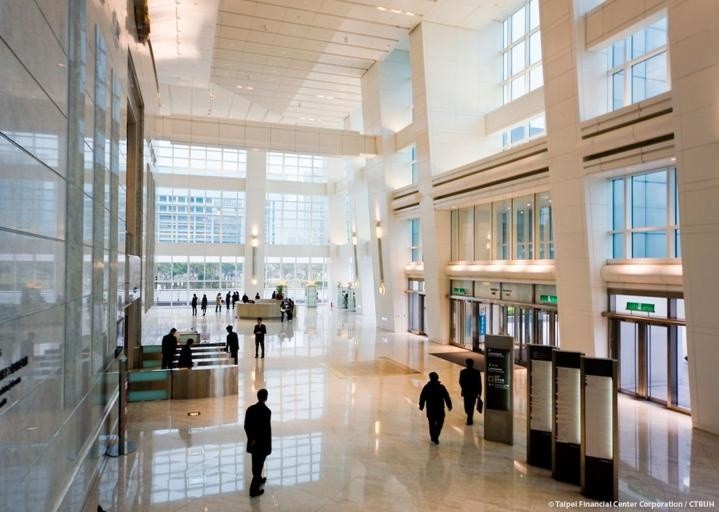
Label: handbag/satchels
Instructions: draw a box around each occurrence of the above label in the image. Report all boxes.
[476,397,483,413]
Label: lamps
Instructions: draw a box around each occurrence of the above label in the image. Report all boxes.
[251,228,258,285]
[352,222,360,289]
[375,203,386,295]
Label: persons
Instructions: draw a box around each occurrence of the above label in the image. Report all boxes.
[459,357,482,426]
[20,332,36,377]
[342,290,348,309]
[418,371,452,446]
[243,389,273,498]
[159,287,296,372]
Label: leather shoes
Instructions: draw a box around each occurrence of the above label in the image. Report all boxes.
[251,489,264,497]
[260,477,266,483]
